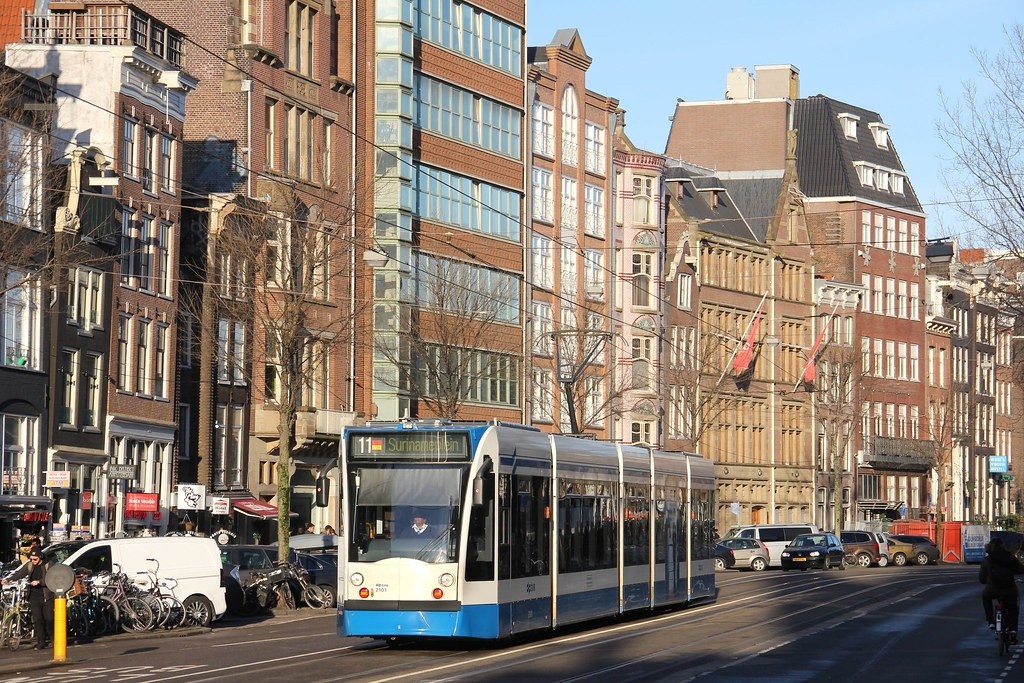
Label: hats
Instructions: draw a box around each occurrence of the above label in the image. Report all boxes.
[30,549,43,560]
[985,538,1005,553]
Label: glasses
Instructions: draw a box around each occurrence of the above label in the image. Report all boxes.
[30,557,39,562]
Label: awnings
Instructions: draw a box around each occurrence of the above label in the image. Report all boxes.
[231,499,299,520]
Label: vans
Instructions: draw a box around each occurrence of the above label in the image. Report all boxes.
[721,524,819,567]
[2,537,228,623]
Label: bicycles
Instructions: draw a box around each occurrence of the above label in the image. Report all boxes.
[989,575,1023,652]
[0,559,187,652]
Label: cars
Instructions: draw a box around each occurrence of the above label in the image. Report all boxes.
[830,530,916,568]
[268,534,346,567]
[979,541,1024,584]
[890,535,940,565]
[715,538,770,574]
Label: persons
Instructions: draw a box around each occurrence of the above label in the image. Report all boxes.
[32,538,42,549]
[325,525,336,535]
[1,547,55,651]
[979,537,1024,643]
[305,523,315,534]
[399,508,437,538]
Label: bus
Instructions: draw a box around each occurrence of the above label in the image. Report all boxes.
[316,329,716,638]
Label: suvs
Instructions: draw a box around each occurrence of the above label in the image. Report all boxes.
[781,533,846,572]
[217,545,338,607]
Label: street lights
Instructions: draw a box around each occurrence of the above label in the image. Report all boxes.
[656,164,693,457]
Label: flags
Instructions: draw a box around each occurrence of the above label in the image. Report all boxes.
[734,303,764,379]
[805,326,826,382]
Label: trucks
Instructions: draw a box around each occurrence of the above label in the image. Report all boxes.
[962,525,990,564]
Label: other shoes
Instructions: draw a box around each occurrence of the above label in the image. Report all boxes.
[988,623,995,629]
[1010,631,1018,645]
[33,640,47,651]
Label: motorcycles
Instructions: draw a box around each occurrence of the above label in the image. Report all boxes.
[245,560,328,611]
